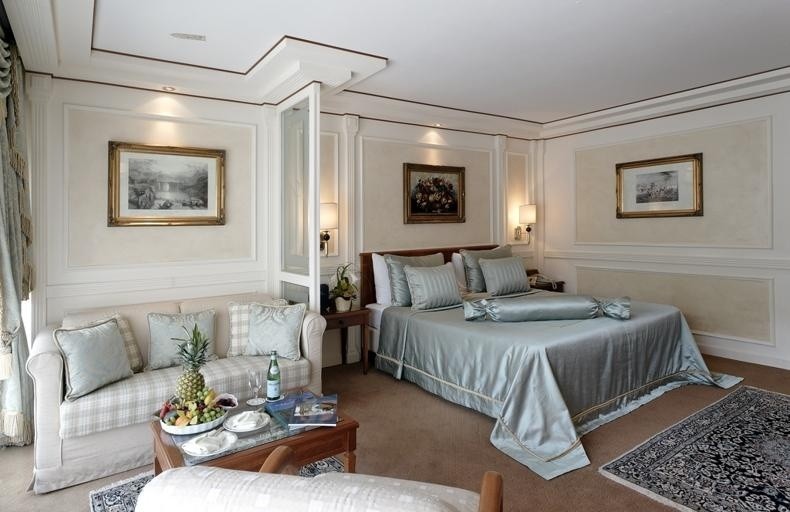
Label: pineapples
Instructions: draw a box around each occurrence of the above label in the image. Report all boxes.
[170,323,209,400]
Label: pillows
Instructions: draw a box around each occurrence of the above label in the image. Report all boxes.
[52,322,132,403]
[458,244,516,294]
[382,252,448,308]
[92,313,145,375]
[450,250,467,292]
[457,289,634,324]
[241,302,310,361]
[478,255,531,297]
[145,308,219,374]
[401,260,463,311]
[223,296,289,356]
[372,253,392,305]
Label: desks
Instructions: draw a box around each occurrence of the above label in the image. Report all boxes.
[530,279,565,293]
[319,308,373,377]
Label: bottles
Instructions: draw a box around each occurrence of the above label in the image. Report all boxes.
[266,349,282,401]
[320,283,330,316]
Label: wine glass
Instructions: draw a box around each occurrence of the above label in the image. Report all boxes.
[246,367,266,406]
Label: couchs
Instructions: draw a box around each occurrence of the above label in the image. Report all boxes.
[25,291,329,494]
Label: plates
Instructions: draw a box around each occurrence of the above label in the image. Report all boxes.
[181,429,237,458]
[265,395,285,402]
[221,411,271,433]
[160,409,229,435]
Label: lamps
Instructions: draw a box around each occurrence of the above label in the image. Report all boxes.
[512,203,539,247]
[319,204,338,256]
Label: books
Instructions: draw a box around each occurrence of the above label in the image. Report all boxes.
[265,392,318,431]
[287,393,338,427]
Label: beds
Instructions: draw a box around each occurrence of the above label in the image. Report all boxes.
[359,244,744,479]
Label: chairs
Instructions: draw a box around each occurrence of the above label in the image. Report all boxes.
[136,438,503,511]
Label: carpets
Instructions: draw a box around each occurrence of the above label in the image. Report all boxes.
[90,453,356,511]
[600,384,790,512]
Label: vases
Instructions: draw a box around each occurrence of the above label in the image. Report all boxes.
[335,299,353,314]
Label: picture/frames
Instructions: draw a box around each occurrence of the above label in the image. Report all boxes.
[106,140,228,230]
[615,151,704,220]
[402,162,466,223]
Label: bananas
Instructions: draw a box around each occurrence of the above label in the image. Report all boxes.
[203,389,216,405]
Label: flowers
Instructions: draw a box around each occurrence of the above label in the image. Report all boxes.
[330,262,360,300]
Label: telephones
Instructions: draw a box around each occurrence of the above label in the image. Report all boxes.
[531,273,555,283]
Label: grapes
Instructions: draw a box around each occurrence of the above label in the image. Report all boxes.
[199,407,227,424]
[196,388,209,400]
